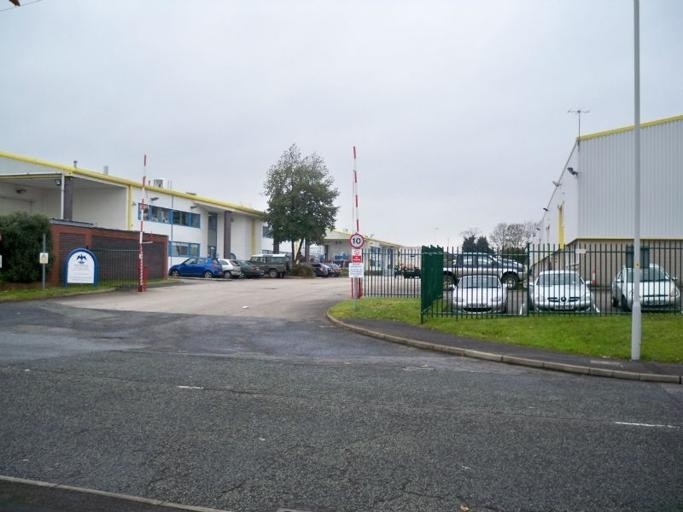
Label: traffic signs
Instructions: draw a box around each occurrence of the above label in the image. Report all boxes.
[348,232,364,249]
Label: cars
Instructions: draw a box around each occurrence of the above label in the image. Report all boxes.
[528,270,595,312]
[609,262,682,312]
[166,253,341,281]
[440,252,526,291]
[446,275,509,314]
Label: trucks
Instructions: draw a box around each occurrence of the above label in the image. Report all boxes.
[396,245,422,279]
